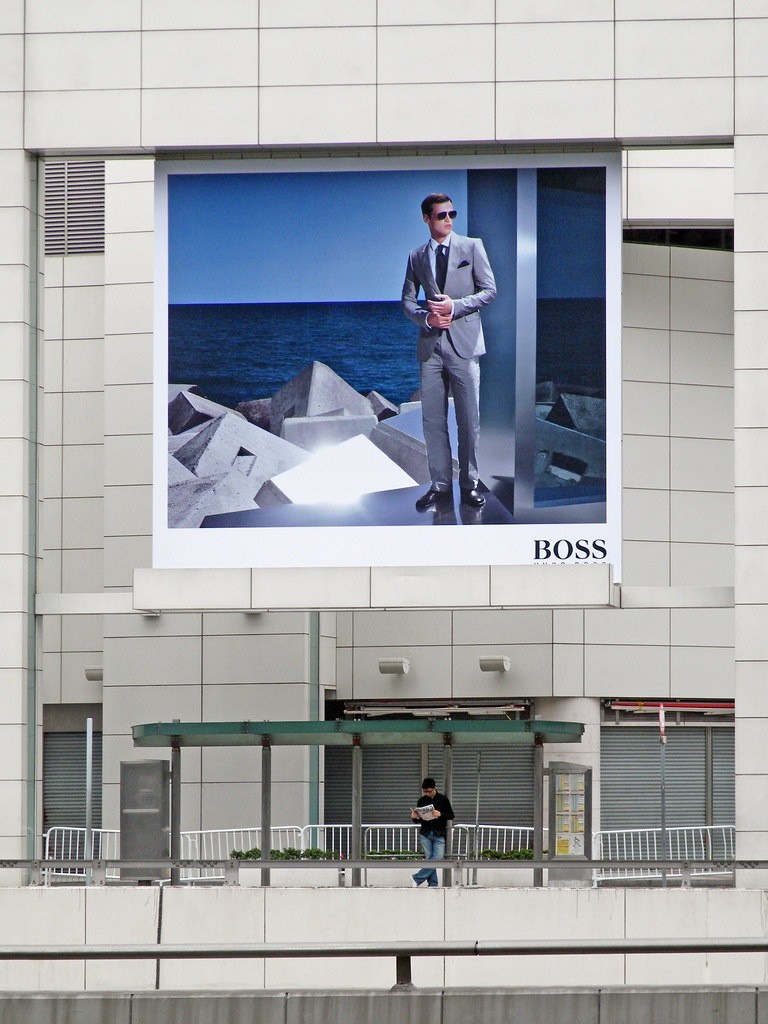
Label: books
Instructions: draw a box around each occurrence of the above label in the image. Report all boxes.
[410,804,438,821]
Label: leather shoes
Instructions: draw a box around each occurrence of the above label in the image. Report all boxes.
[417,490,449,507]
[462,487,486,506]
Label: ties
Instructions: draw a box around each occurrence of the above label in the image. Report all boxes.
[435,244,448,294]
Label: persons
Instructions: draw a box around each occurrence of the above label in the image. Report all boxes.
[409,777,455,887]
[402,192,498,511]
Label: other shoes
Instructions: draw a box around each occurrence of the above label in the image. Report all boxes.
[411,875,418,888]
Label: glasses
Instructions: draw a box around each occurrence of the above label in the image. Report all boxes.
[423,790,434,793]
[429,210,457,220]
